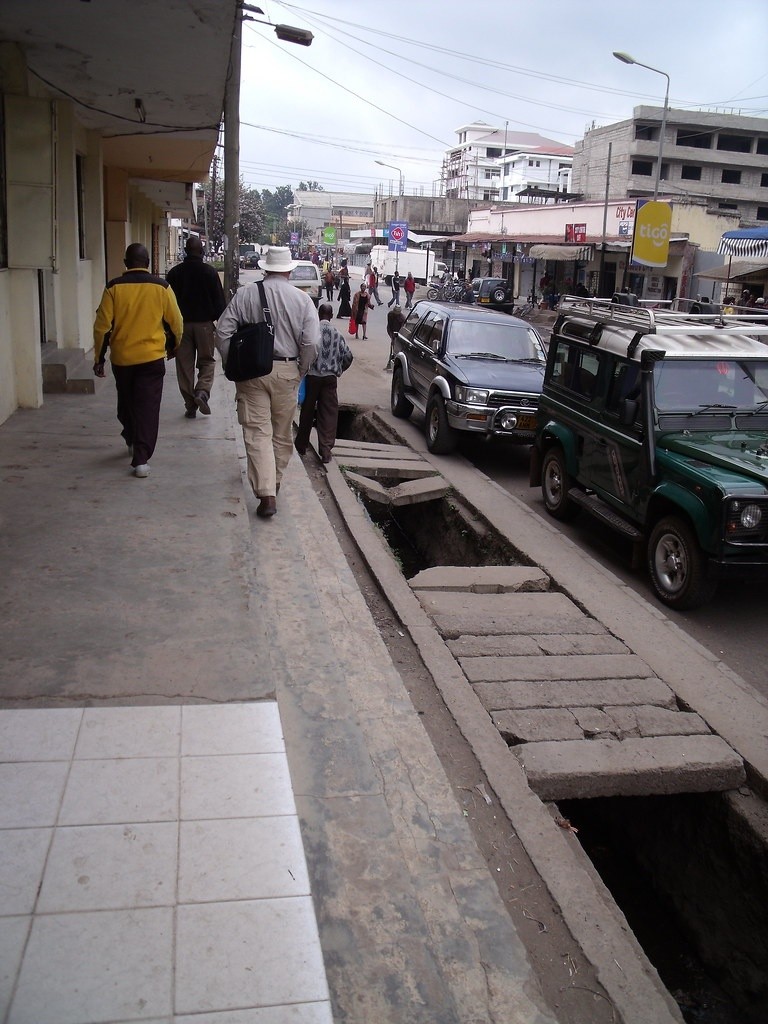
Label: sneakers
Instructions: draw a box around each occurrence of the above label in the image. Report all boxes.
[134,463,150,478]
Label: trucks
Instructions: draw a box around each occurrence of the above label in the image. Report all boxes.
[370,245,449,287]
[239,244,255,268]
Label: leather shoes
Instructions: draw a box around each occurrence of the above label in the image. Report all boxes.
[256,496,277,516]
[185,392,211,419]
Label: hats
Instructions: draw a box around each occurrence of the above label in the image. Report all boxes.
[408,272,411,275]
[258,246,298,272]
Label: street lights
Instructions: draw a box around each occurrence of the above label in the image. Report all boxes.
[613,51,670,308]
[224,15,314,309]
[374,161,401,271]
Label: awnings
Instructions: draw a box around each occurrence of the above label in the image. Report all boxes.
[529,245,594,261]
[716,226,768,258]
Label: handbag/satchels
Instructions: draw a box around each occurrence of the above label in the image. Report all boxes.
[224,281,275,382]
[348,319,357,334]
[298,376,306,406]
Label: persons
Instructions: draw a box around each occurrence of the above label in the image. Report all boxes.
[388,271,400,307]
[92,243,183,478]
[722,290,768,315]
[294,304,353,463]
[337,278,352,319]
[364,262,384,307]
[291,247,324,269]
[613,283,641,299]
[670,283,677,300]
[214,247,321,517]
[383,305,407,370]
[404,272,415,308]
[165,236,227,419]
[538,272,596,311]
[325,254,349,302]
[351,283,374,340]
[439,263,480,304]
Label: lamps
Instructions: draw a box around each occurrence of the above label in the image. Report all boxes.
[242,15,314,47]
[135,98,147,123]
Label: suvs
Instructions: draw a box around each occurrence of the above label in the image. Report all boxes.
[244,251,260,269]
[530,289,768,611]
[389,299,560,455]
[287,259,323,308]
[470,277,514,315]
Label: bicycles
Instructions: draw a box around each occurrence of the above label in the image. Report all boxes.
[427,279,471,303]
[514,295,535,318]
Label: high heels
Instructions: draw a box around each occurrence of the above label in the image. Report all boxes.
[356,333,359,339]
[363,336,368,340]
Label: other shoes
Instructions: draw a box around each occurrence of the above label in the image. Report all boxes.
[322,455,332,463]
[294,443,306,455]
[378,303,383,306]
